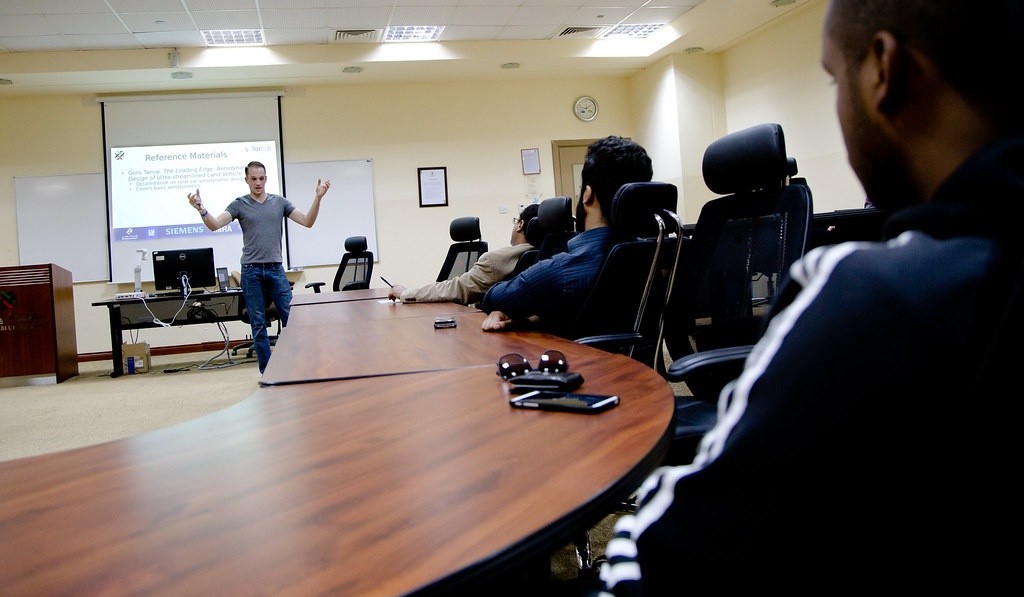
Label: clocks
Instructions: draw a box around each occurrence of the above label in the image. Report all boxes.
[574,96,599,122]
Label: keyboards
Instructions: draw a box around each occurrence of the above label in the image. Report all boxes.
[154,290,205,298]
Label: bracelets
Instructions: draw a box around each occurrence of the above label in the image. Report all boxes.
[201,210,208,221]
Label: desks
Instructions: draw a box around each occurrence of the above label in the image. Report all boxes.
[92,286,293,378]
[0,290,676,597]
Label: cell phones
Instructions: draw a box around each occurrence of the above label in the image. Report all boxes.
[434,316,457,328]
[510,391,621,415]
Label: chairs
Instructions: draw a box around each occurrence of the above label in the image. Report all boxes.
[503,123,899,585]
[436,217,488,283]
[305,237,374,294]
[232,271,294,358]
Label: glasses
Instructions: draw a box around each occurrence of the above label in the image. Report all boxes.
[496,349,568,379]
[512,218,519,225]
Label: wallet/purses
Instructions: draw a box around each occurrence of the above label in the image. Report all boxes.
[508,371,585,394]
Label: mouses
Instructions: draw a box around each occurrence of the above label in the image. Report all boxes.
[149,293,155,297]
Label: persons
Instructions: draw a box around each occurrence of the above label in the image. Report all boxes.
[388,204,540,304]
[188,161,330,376]
[598,0,1024,597]
[481,135,654,331]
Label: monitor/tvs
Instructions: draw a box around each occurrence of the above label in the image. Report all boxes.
[153,248,216,299]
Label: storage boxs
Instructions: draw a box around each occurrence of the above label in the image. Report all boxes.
[121,341,151,375]
